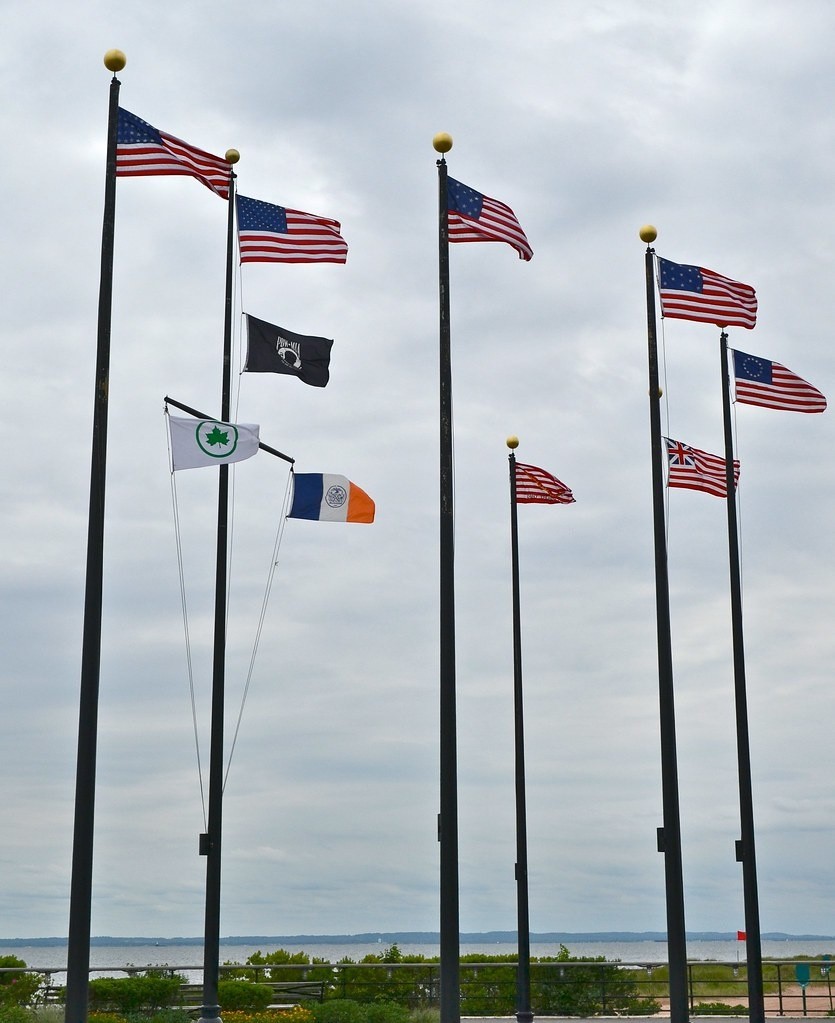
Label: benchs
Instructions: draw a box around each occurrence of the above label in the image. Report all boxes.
[34,986,65,1008]
[147,980,324,1011]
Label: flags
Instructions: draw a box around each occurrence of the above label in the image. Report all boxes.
[664,437,741,499]
[235,194,349,266]
[164,405,260,475]
[731,349,828,414]
[737,931,747,941]
[239,312,334,388]
[657,257,758,330]
[115,106,231,200]
[286,466,376,525]
[446,175,534,262]
[515,462,577,504]
